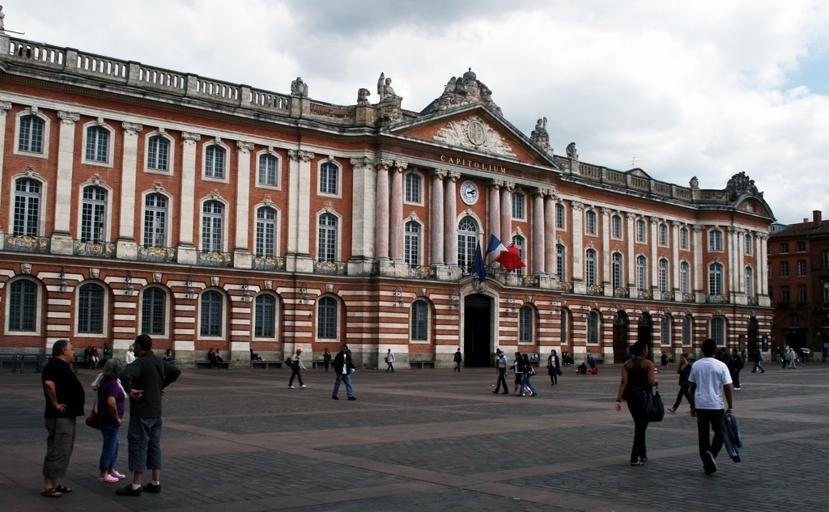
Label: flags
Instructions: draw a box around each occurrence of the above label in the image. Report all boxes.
[482,234,508,266]
[496,243,527,272]
[475,241,487,284]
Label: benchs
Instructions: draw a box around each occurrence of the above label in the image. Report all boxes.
[254,359,283,369]
[196,359,233,366]
[0,351,109,377]
[409,360,434,369]
[312,358,334,368]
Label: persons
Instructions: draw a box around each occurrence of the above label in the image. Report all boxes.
[96,357,127,484]
[716,337,801,390]
[660,350,670,369]
[215,348,224,363]
[323,348,331,372]
[453,348,462,372]
[666,353,696,413]
[577,362,587,375]
[588,365,599,376]
[207,347,217,368]
[492,348,509,394]
[546,349,561,386]
[508,351,524,394]
[384,348,395,373]
[165,348,174,362]
[563,350,574,364]
[687,338,734,476]
[332,344,358,400]
[125,344,136,368]
[287,348,307,389]
[115,334,182,496]
[517,354,538,397]
[251,348,262,361]
[85,342,112,368]
[40,339,86,498]
[587,350,595,364]
[614,340,658,467]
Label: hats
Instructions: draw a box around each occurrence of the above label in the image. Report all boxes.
[296,349,302,352]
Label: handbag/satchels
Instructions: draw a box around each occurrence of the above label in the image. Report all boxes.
[285,357,292,366]
[641,383,664,421]
[86,408,101,430]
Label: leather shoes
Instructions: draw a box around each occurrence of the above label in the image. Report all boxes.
[332,395,357,400]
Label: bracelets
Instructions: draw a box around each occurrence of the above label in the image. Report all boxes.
[616,399,621,403]
[726,408,734,413]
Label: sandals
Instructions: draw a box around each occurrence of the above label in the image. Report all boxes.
[40,484,72,497]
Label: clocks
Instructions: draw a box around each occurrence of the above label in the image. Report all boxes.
[459,179,480,206]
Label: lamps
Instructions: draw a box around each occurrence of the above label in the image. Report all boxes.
[393,286,404,309]
[299,284,311,305]
[122,271,135,298]
[56,265,69,296]
[240,280,253,304]
[505,294,517,316]
[184,275,196,302]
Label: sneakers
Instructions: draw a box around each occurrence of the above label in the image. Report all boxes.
[288,385,306,389]
[705,450,718,472]
[96,470,160,495]
[631,451,648,465]
